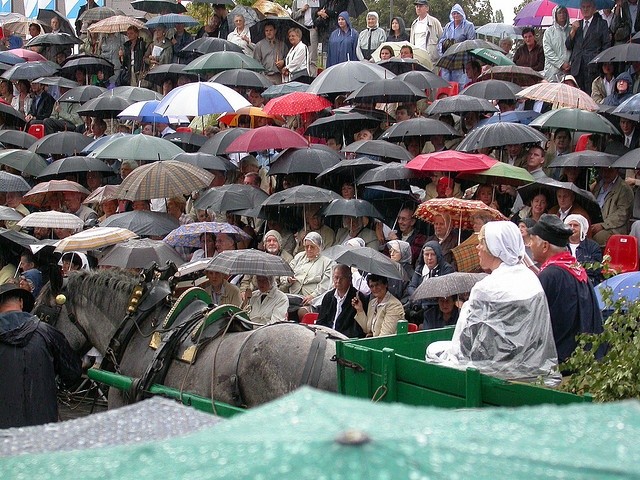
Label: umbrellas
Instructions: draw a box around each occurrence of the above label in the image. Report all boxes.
[205,248,295,299]
[305,53,398,93]
[252,0,289,18]
[627,31,640,43]
[82,184,122,204]
[423,94,500,114]
[129,0,188,14]
[223,123,312,153]
[469,110,543,127]
[304,112,383,157]
[457,160,536,204]
[404,150,499,189]
[356,161,419,191]
[470,48,517,67]
[435,39,508,70]
[153,73,253,135]
[513,0,585,27]
[98,210,180,236]
[454,112,548,162]
[16,211,85,239]
[22,180,91,208]
[215,106,274,127]
[262,183,346,233]
[250,15,311,62]
[370,41,433,71]
[515,179,605,225]
[36,148,114,183]
[609,148,640,169]
[0,12,26,23]
[0,386,640,480]
[31,75,82,89]
[527,98,623,153]
[315,156,389,198]
[267,134,347,176]
[79,135,114,153]
[456,71,525,100]
[551,0,616,21]
[547,143,621,191]
[393,65,455,90]
[476,22,526,40]
[7,48,48,61]
[116,92,190,136]
[587,35,640,73]
[593,271,640,311]
[313,197,385,218]
[378,60,435,75]
[53,225,140,254]
[262,92,334,127]
[85,126,186,160]
[378,109,465,155]
[164,125,210,147]
[0,127,39,148]
[77,6,116,20]
[197,125,253,154]
[0,50,27,66]
[57,80,106,102]
[1,17,45,35]
[611,92,640,122]
[413,196,511,231]
[144,63,205,88]
[61,55,114,86]
[111,153,215,213]
[37,8,75,36]
[98,80,165,102]
[77,89,130,134]
[476,64,544,86]
[194,182,270,210]
[162,221,253,257]
[226,4,266,32]
[409,270,492,315]
[0,226,40,250]
[181,44,266,73]
[261,82,311,96]
[0,101,27,126]
[59,53,115,67]
[451,233,483,273]
[320,0,367,18]
[0,59,63,80]
[0,205,24,221]
[515,82,601,112]
[179,31,245,55]
[28,130,96,154]
[321,246,410,303]
[86,15,148,61]
[170,151,240,172]
[343,72,427,128]
[192,0,235,27]
[206,61,276,89]
[341,138,416,162]
[0,170,32,191]
[24,31,85,47]
[0,149,50,177]
[143,13,200,39]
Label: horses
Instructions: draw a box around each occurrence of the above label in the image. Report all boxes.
[29,262,350,410]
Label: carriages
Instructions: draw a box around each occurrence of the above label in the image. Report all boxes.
[29,261,592,417]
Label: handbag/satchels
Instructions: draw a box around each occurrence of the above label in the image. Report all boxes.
[115,68,130,87]
[290,68,309,81]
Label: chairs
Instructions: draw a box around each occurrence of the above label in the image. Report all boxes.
[427,81,460,105]
[301,313,320,324]
[407,323,419,332]
[574,133,591,152]
[175,126,193,132]
[28,124,45,139]
[601,234,640,275]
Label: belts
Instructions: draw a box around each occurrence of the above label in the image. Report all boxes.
[305,26,315,29]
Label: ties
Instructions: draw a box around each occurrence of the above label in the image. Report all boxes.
[583,21,589,39]
[626,138,629,148]
[552,154,562,179]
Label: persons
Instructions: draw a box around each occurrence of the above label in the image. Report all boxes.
[0,191,7,206]
[428,88,449,102]
[19,251,48,283]
[55,228,72,239]
[5,191,30,217]
[336,215,380,252]
[246,89,264,109]
[119,25,148,86]
[166,195,195,225]
[340,180,355,199]
[162,76,176,96]
[316,1,348,51]
[143,23,173,66]
[28,23,41,39]
[215,232,238,253]
[425,220,562,380]
[424,171,463,200]
[591,62,617,102]
[226,14,252,43]
[48,193,65,212]
[0,79,14,100]
[4,35,23,51]
[409,0,444,64]
[547,188,592,239]
[378,46,395,60]
[24,80,57,122]
[196,209,211,222]
[251,229,293,294]
[177,74,192,86]
[436,3,476,91]
[564,0,611,95]
[626,61,640,94]
[4,165,15,174]
[11,80,32,113]
[282,27,311,83]
[599,9,613,26]
[525,145,550,182]
[237,115,251,128]
[97,238,184,270]
[527,213,608,377]
[239,155,276,193]
[464,61,483,87]
[476,184,499,210]
[502,144,527,168]
[64,192,99,230]
[87,171,104,190]
[30,86,85,136]
[287,232,337,321]
[430,134,448,152]
[157,121,177,136]
[0,252,17,283]
[585,134,604,152]
[120,159,138,181]
[211,4,231,39]
[398,208,427,269]
[75,67,89,85]
[562,215,603,287]
[560,168,588,190]
[591,167,635,254]
[141,124,157,136]
[614,117,640,152]
[75,0,100,37]
[472,215,494,232]
[387,239,415,279]
[497,98,516,111]
[386,16,411,42]
[294,204,336,255]
[399,44,414,59]
[226,210,256,236]
[282,174,297,189]
[95,68,108,87]
[1,112,20,130]
[190,232,219,261]
[118,123,132,134]
[542,5,575,82]
[33,227,51,239]
[435,175,456,199]
[351,273,405,337]
[518,217,538,261]
[97,199,119,223]
[623,169,640,219]
[252,23,289,84]
[19,269,45,298]
[477,147,495,158]
[354,129,373,142]
[90,117,108,139]
[423,295,461,329]
[291,1,321,80]
[545,128,572,179]
[50,17,63,33]
[355,11,388,60]
[407,241,455,300]
[326,134,343,152]
[521,193,562,225]
[205,270,243,308]
[425,213,459,242]
[499,37,515,60]
[0,283,84,428]
[396,104,414,122]
[55,52,67,65]
[560,75,579,89]
[132,200,148,210]
[61,253,83,277]
[195,11,222,39]
[611,0,640,43]
[513,28,545,71]
[602,72,636,107]
[240,274,289,324]
[267,213,294,254]
[243,172,262,189]
[317,264,368,339]
[326,10,360,67]
[170,23,194,65]
[403,136,420,154]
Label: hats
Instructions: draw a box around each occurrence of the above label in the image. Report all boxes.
[413,0,428,5]
[0,283,35,313]
[526,213,574,247]
